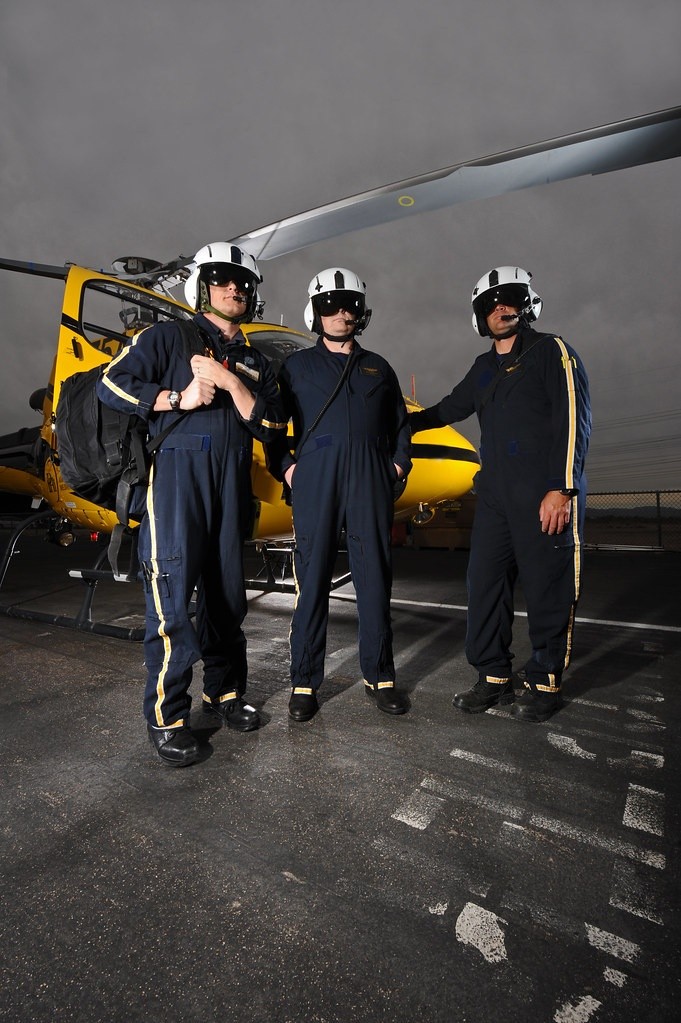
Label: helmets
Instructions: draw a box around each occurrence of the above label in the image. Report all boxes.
[470,265,543,338]
[184,241,264,318]
[304,267,372,342]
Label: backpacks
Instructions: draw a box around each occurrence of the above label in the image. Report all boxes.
[55,317,220,522]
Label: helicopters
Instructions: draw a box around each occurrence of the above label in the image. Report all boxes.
[0,104,681,641]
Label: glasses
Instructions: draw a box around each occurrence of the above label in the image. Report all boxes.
[311,291,365,318]
[472,285,525,318]
[200,265,258,297]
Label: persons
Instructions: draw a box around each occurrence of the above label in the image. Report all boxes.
[95,241,276,765]
[408,266,592,720]
[261,268,412,720]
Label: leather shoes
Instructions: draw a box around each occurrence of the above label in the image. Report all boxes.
[509,684,563,723]
[147,721,202,767]
[287,680,317,721]
[451,676,516,714]
[364,680,408,715]
[202,691,261,732]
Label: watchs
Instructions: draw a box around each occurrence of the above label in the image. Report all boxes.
[167,389,182,412]
[560,489,573,496]
